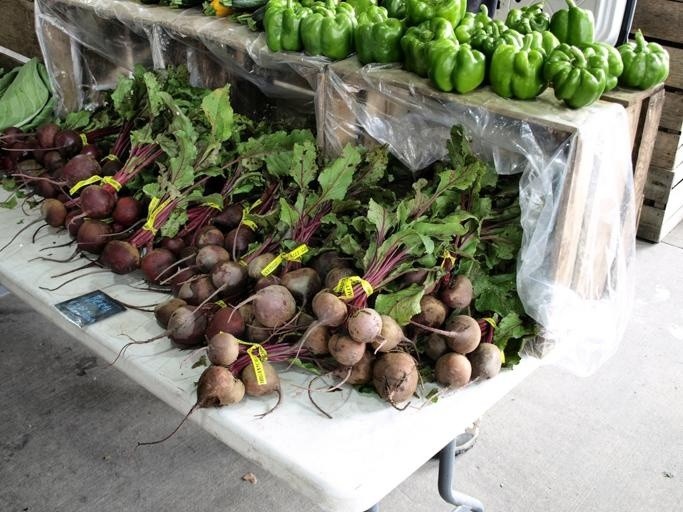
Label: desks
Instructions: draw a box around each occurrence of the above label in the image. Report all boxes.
[1,181,535,512]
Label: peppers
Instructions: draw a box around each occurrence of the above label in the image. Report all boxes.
[263,0,669,110]
